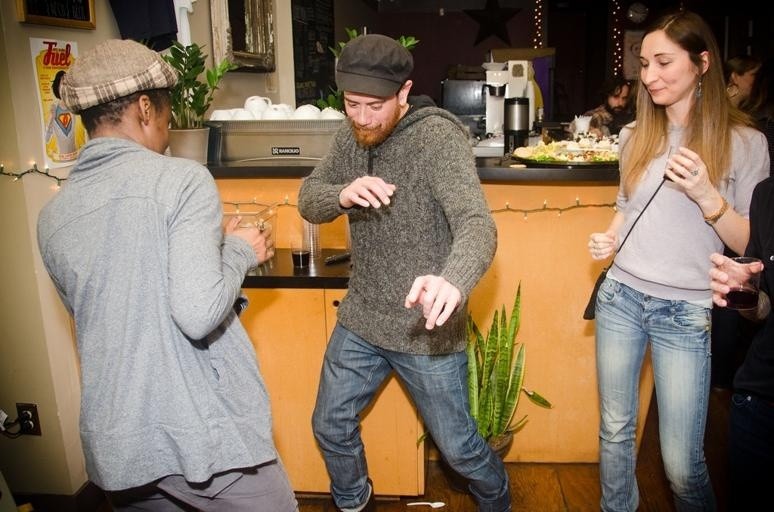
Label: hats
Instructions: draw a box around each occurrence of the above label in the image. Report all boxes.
[335,33,417,99]
[61,37,180,114]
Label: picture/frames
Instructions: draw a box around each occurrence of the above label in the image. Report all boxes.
[209,0,277,77]
[15,0,96,33]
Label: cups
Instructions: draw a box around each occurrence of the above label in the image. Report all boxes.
[291,238,313,272]
[207,95,346,119]
[714,256,766,314]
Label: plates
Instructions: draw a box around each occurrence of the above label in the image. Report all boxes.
[510,153,620,165]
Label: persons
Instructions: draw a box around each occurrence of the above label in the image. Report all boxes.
[708,175,774,512]
[588,12,770,512]
[581,52,774,176]
[296,34,498,512]
[38,39,300,512]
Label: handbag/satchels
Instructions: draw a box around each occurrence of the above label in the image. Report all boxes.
[583,117,705,322]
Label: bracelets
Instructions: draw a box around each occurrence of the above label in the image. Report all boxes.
[704,197,730,225]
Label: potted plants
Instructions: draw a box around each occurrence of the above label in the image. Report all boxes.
[411,280,553,496]
[159,41,236,169]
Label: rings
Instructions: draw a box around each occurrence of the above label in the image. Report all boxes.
[256,220,265,232]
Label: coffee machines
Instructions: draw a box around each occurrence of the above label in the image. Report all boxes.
[473,59,545,158]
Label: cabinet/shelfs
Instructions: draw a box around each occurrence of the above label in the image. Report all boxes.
[225,287,431,502]
